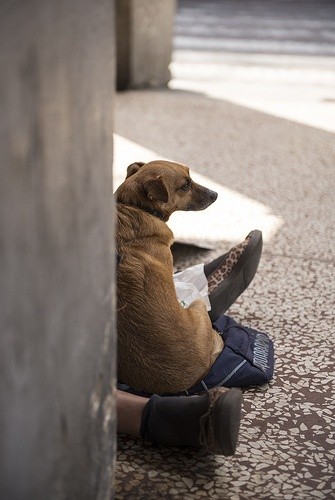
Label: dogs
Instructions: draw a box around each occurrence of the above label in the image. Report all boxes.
[115,160,224,395]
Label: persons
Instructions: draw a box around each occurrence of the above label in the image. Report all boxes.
[116,230,263,456]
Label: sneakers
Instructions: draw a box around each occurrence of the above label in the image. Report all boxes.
[201,384,244,457]
[207,229,262,322]
